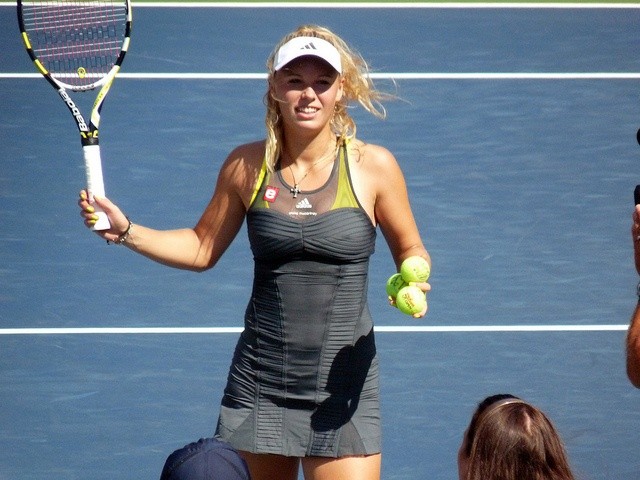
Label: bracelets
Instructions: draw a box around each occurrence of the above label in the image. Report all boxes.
[114,217,134,246]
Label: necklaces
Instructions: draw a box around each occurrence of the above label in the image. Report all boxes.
[282,146,337,198]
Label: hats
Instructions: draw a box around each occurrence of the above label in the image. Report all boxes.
[272,36,342,75]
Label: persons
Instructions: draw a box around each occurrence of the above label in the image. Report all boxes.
[455,393,577,479]
[624,127,640,391]
[72,25,435,480]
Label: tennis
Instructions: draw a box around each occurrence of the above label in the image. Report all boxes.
[396,285,425,314]
[386,273,406,299]
[400,255,430,285]
